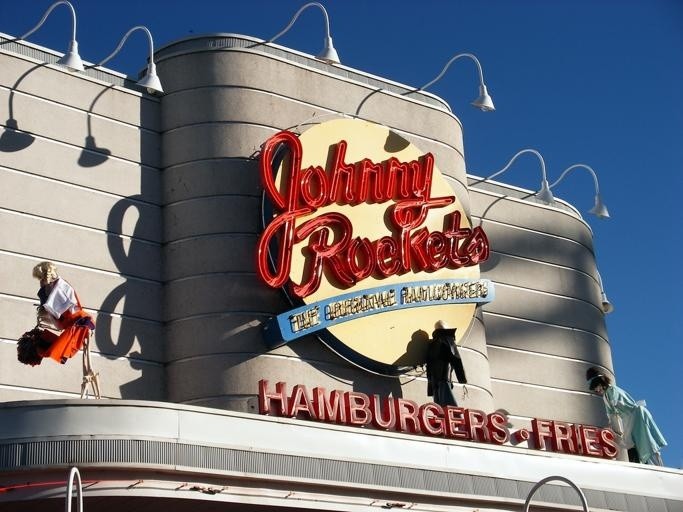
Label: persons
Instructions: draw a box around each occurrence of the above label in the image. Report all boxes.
[587,366,668,464]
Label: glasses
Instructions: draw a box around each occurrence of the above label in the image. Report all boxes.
[268,2,341,65]
[536,164,610,219]
[522,476,587,511]
[96,24,164,96]
[65,466,83,512]
[19,1,86,72]
[487,149,556,205]
[598,270,613,314]
[420,53,496,113]
